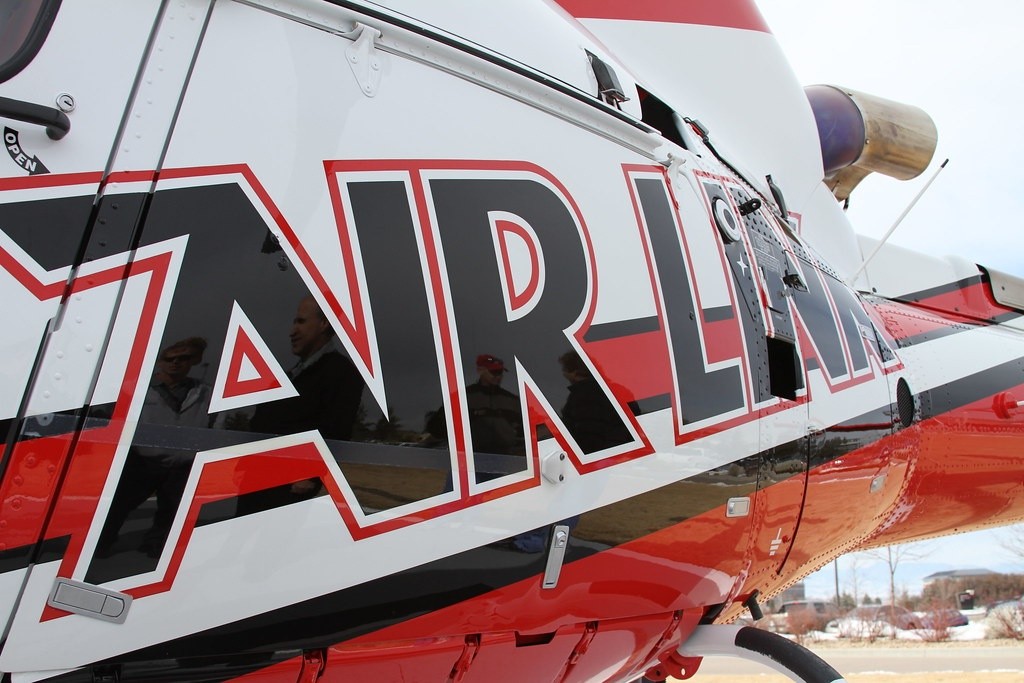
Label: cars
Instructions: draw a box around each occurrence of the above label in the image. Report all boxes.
[826,604,922,636]
[924,610,969,628]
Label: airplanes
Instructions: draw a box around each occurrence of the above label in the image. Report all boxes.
[1,1,1022,683]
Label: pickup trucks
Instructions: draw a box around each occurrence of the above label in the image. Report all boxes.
[765,599,844,635]
